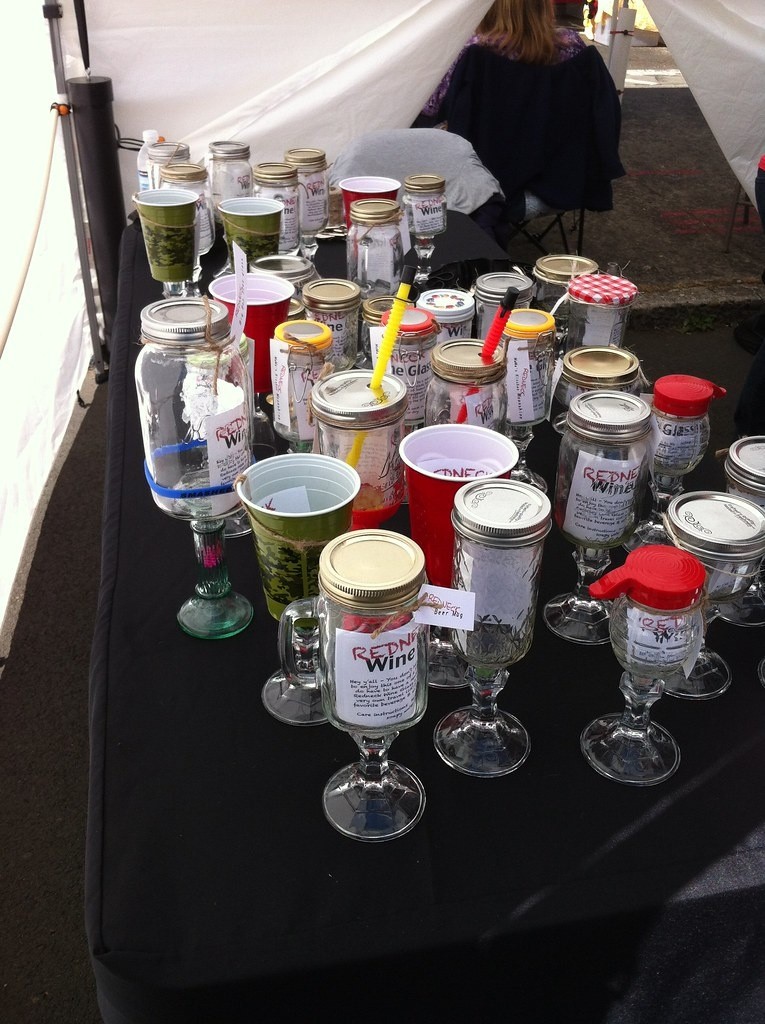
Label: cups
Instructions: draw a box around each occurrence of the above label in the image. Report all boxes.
[338,176,402,257]
[132,188,200,281]
[399,424,519,587]
[218,197,285,271]
[210,272,294,393]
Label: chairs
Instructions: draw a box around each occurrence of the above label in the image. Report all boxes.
[437,44,627,257]
[329,126,508,249]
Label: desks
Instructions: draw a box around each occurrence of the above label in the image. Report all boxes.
[83,210,765,1024]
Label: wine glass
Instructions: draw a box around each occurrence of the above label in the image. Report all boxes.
[134,142,765,842]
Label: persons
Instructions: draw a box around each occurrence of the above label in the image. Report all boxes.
[419,0,588,241]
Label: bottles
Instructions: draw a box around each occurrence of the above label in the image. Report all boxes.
[136,130,159,192]
[346,199,404,298]
